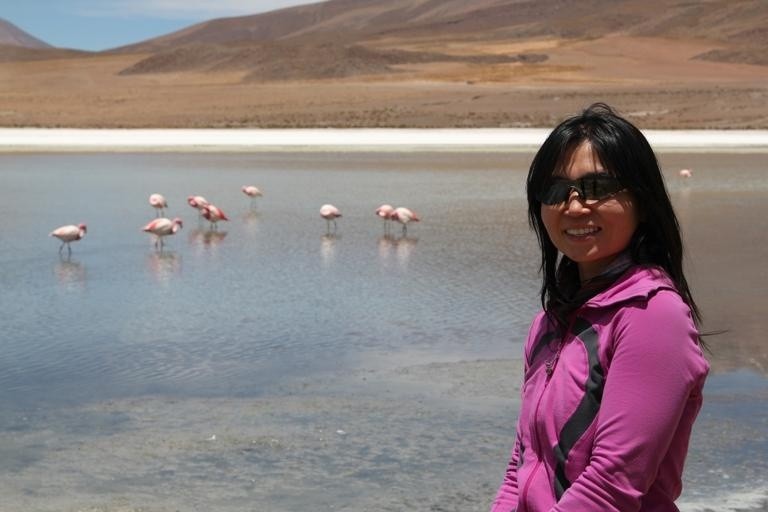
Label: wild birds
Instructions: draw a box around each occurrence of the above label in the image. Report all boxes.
[140,218,183,247]
[49,223,87,253]
[390,207,420,233]
[201,205,230,228]
[242,185,262,207]
[375,204,394,229]
[680,169,691,178]
[148,193,168,217]
[320,204,343,228]
[188,195,211,214]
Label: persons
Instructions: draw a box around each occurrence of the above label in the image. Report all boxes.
[492,99,711,512]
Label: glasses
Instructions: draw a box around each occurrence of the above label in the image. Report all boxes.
[533,172,624,205]
